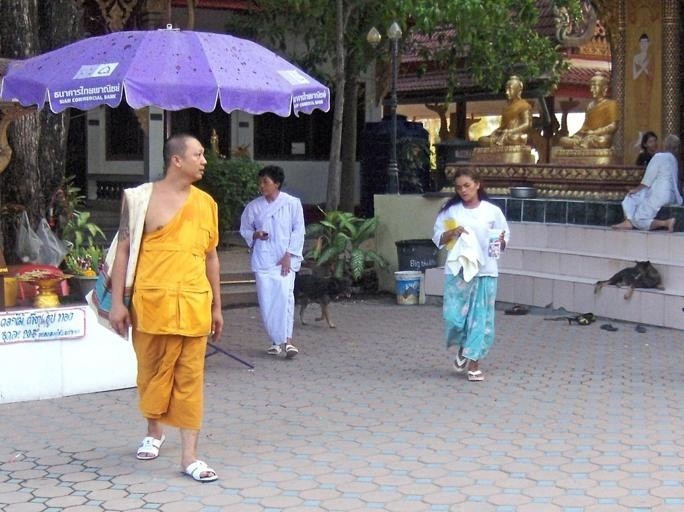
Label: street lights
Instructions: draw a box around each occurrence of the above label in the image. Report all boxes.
[365,21,403,195]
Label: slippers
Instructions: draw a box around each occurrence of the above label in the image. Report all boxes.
[266,345,282,355]
[178,460,218,481]
[137,434,165,460]
[286,344,298,357]
[505,306,528,314]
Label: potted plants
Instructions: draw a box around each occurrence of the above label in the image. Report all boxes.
[319,211,389,294]
[306,212,359,293]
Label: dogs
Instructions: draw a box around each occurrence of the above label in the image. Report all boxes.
[594,260,665,300]
[294,271,353,328]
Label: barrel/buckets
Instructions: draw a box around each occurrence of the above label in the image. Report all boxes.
[393,270,422,306]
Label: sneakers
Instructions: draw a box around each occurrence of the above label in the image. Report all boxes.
[635,323,646,332]
[600,324,618,331]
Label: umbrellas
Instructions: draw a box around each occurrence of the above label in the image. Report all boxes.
[0,23,331,142]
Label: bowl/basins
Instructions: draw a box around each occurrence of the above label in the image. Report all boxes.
[510,187,536,199]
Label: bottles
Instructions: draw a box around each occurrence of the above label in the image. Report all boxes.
[488,230,501,261]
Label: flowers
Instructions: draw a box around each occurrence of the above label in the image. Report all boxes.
[65,245,109,277]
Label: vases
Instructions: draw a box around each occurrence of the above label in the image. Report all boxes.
[77,275,98,304]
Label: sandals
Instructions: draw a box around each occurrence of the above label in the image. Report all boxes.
[467,370,484,381]
[454,349,467,371]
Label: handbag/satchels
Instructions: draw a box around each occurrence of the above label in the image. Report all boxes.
[85,181,153,340]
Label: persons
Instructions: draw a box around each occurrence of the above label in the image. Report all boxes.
[632,34,655,147]
[612,135,684,232]
[558,71,622,149]
[636,131,658,166]
[432,167,511,381]
[239,166,306,357]
[108,133,223,482]
[477,74,533,145]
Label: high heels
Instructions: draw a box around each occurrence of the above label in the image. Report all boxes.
[567,313,596,325]
